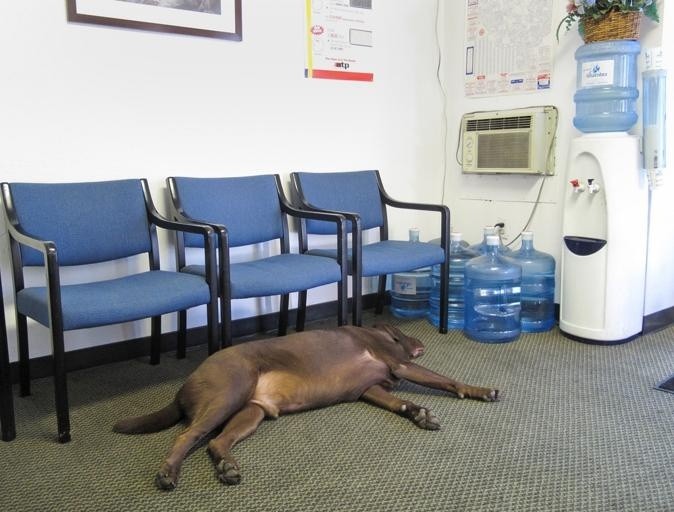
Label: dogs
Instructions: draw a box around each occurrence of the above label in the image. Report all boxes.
[111,323,503,492]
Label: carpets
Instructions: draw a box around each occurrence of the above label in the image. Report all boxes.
[653,373,674,394]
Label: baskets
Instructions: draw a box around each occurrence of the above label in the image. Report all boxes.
[585,11,640,43]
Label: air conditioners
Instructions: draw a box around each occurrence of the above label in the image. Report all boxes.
[460,106,555,175]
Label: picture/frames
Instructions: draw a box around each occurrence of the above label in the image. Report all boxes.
[66,0,242,42]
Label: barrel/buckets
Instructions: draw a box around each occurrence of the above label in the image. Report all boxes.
[391,225,556,342]
[572,40,642,132]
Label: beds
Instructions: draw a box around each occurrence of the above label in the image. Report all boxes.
[166,174,348,360]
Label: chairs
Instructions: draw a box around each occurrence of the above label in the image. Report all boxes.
[1,178,220,443]
[291,170,450,331]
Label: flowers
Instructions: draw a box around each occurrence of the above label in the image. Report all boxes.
[557,0,659,43]
